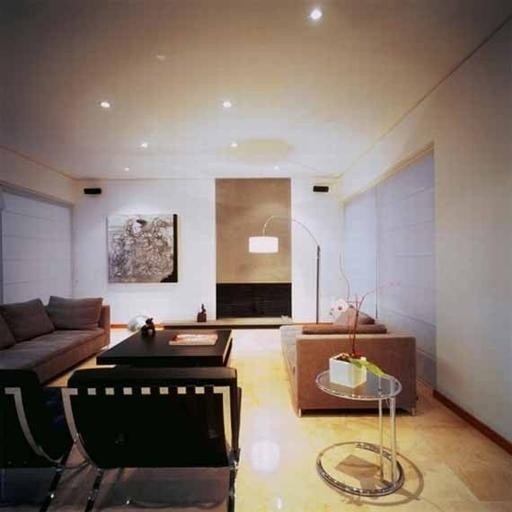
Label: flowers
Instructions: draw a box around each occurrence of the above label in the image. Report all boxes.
[328,282,382,354]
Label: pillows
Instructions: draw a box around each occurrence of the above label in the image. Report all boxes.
[0,314,17,350]
[45,296,104,330]
[301,322,387,334]
[333,306,375,325]
[0,297,55,343]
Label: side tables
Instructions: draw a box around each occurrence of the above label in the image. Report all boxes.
[315,368,405,497]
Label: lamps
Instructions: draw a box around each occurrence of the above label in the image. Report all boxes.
[249,214,320,323]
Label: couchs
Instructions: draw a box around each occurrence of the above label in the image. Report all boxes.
[279,310,416,417]
[0,304,111,386]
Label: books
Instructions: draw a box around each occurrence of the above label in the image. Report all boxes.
[168,334,218,346]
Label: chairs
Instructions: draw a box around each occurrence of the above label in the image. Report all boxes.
[61,365,242,512]
[0,367,74,512]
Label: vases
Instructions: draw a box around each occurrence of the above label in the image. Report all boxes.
[328,351,368,389]
